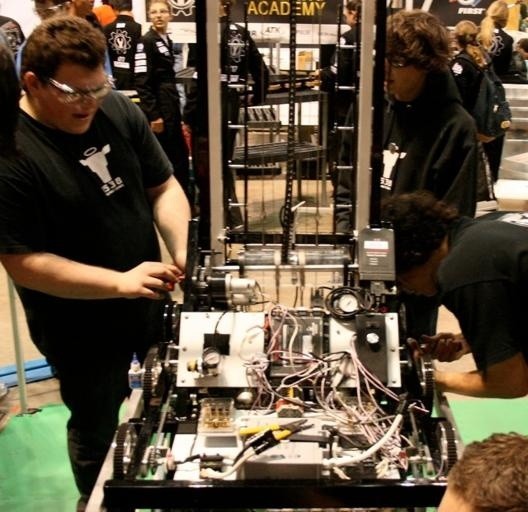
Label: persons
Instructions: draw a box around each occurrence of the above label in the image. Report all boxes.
[1,15,192,511]
[435,431,527,511]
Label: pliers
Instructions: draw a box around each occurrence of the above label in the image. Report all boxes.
[238,419,314,441]
